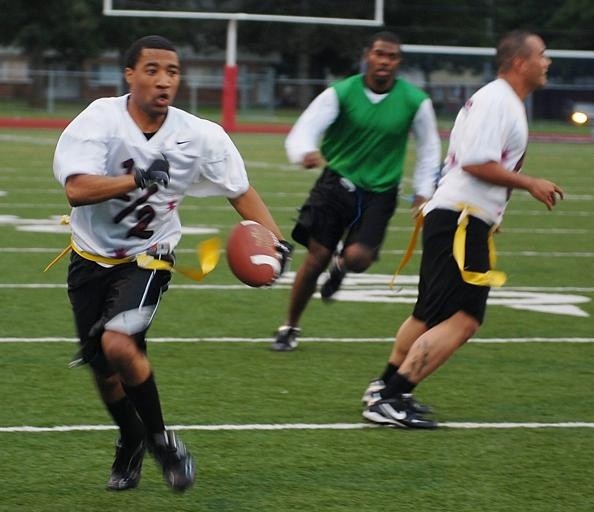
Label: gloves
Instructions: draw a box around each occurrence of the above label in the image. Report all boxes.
[134,158,172,190]
[274,238,293,275]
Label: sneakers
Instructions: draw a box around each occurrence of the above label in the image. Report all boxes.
[361,379,437,429]
[107,425,146,492]
[148,428,194,493]
[322,252,345,299]
[273,324,301,351]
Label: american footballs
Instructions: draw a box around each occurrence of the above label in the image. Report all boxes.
[227,219,282,288]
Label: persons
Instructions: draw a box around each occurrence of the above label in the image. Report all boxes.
[265,30,443,352]
[361,29,565,432]
[53,34,293,493]
